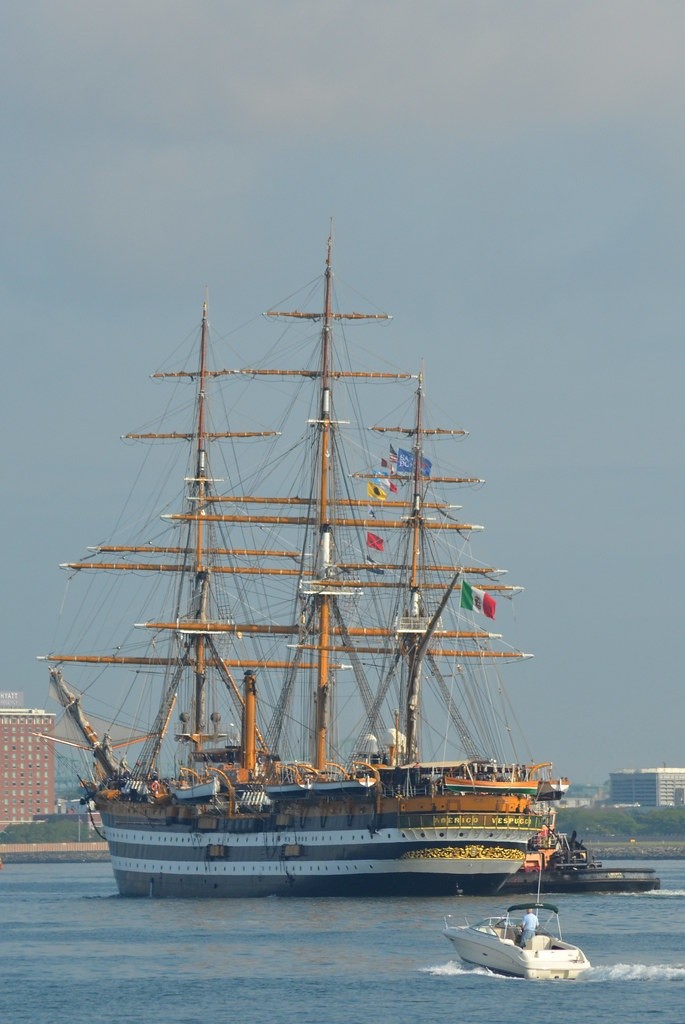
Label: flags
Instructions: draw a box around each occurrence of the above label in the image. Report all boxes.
[390,443,395,459]
[366,506,373,515]
[374,472,398,493]
[461,580,496,621]
[381,457,394,472]
[366,556,383,575]
[367,481,387,501]
[397,449,432,477]
[366,533,386,551]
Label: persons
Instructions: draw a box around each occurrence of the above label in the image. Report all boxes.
[519,909,540,948]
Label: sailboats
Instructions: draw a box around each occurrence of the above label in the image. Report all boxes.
[32,213,572,891]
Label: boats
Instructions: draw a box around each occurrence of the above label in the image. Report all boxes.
[501,834,662,894]
[440,860,593,983]
